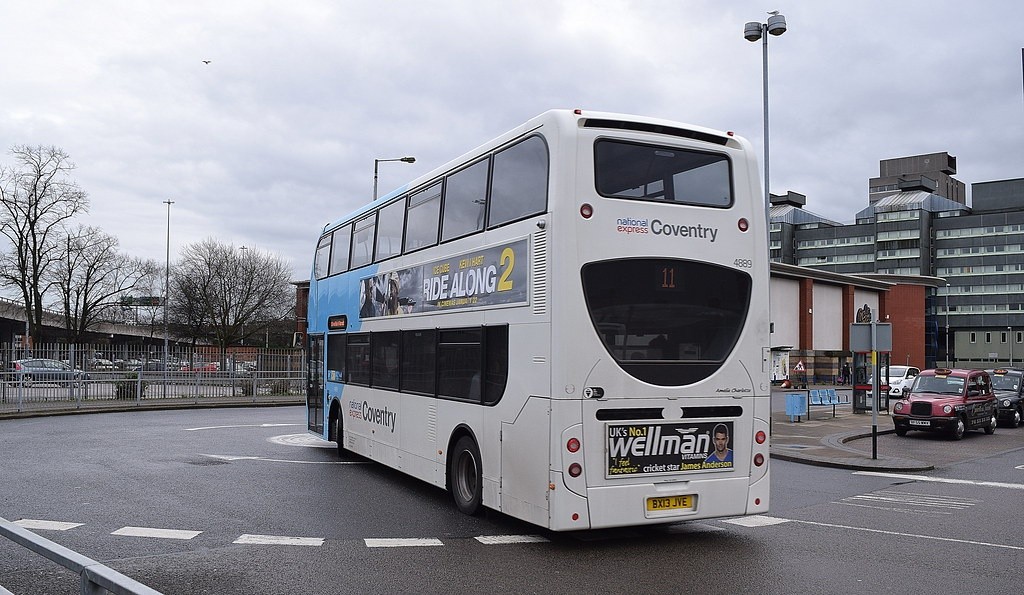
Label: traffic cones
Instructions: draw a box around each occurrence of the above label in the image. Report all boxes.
[780,379,793,389]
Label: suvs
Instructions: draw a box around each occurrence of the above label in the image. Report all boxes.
[980,368,1024,428]
[893,367,997,440]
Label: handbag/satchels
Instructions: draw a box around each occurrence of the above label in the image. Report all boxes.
[837,377,843,383]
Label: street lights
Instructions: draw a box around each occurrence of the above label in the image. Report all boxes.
[744,10,789,258]
[162,198,175,399]
[372,157,417,202]
[946,283,951,369]
[1007,326,1012,366]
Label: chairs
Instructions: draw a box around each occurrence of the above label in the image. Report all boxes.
[810,388,851,405]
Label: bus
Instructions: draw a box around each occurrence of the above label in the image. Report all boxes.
[291,107,769,533]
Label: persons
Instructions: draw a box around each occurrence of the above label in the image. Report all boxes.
[837,362,850,386]
[704,424,733,464]
[360,272,412,318]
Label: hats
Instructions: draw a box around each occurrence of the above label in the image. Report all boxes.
[389,272,400,294]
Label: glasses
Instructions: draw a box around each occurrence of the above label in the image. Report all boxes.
[366,286,373,292]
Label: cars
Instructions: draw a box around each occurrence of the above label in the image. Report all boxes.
[8,359,91,389]
[865,365,920,400]
[61,358,257,376]
